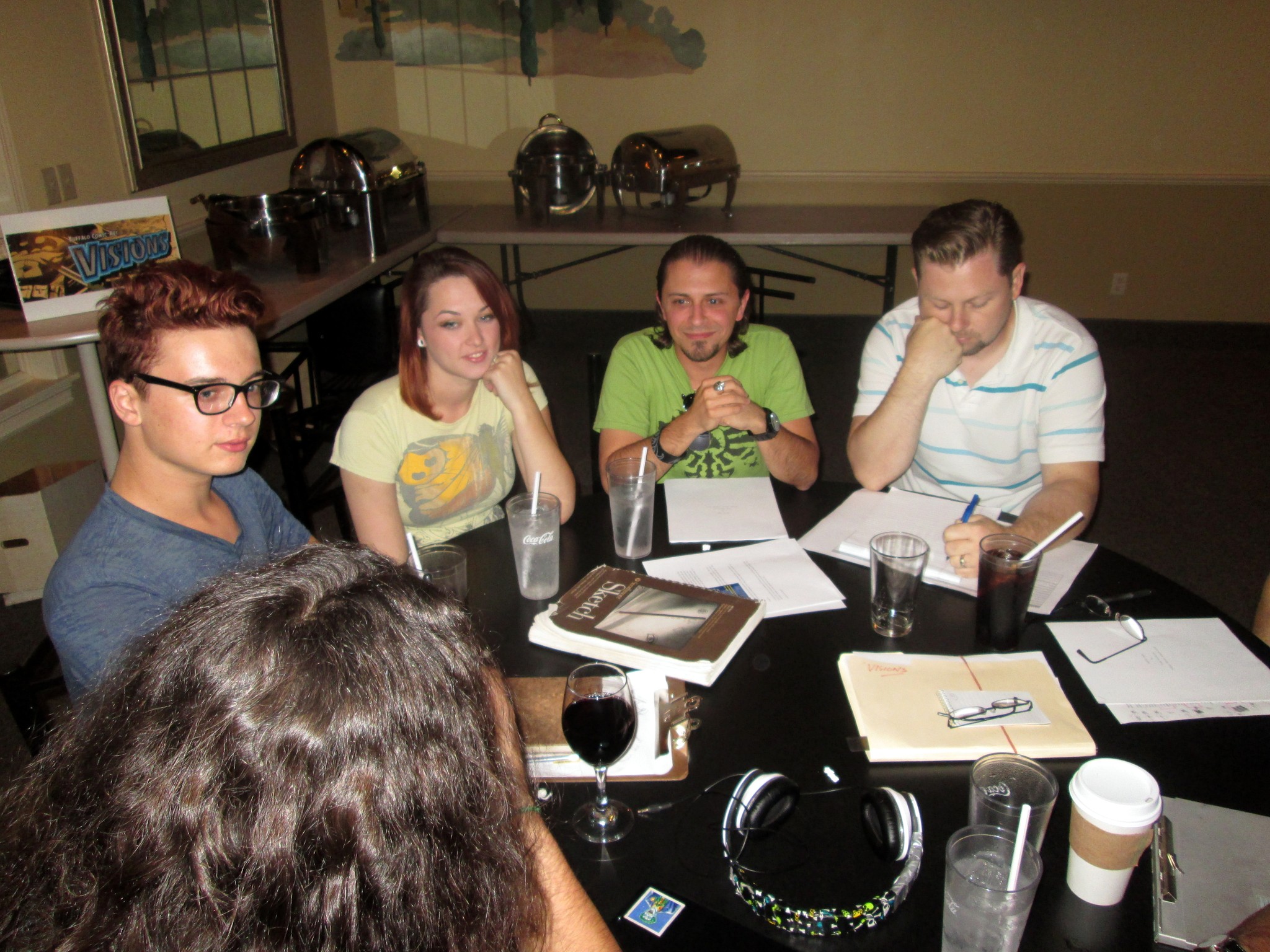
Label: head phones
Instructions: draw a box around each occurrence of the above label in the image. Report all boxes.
[720,767,924,935]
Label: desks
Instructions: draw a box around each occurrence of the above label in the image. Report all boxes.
[426,480,1270,952]
[436,203,945,332]
[0,204,471,479]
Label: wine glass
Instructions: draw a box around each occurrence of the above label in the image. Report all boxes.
[561,662,637,843]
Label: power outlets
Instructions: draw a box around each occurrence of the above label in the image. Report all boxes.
[1110,272,1129,298]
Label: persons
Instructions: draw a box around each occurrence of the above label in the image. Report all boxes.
[847,199,1107,578]
[593,233,820,492]
[328,247,576,568]
[0,537,622,952]
[1253,575,1270,646]
[1192,901,1270,952]
[39,259,321,711]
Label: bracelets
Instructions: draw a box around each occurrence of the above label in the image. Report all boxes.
[651,422,687,464]
[514,804,544,816]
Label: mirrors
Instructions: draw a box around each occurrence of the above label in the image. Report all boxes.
[92,0,300,197]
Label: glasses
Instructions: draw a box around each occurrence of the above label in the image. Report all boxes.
[126,369,283,415]
[1044,595,1147,663]
[938,696,1034,729]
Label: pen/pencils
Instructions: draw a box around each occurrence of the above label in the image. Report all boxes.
[1098,590,1152,603]
[943,494,981,562]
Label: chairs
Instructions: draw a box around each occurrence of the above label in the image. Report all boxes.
[267,341,385,542]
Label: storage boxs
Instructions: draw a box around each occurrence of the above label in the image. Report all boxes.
[0,460,107,606]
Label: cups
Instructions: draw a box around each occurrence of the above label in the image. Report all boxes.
[967,752,1059,853]
[1066,758,1162,906]
[940,824,1044,952]
[869,532,928,637]
[975,534,1042,647]
[605,457,656,559]
[506,492,561,600]
[406,542,467,606]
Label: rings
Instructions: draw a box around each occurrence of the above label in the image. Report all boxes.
[713,381,724,392]
[960,554,966,568]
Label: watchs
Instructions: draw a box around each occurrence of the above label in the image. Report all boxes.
[747,407,780,442]
[1198,935,1241,952]
